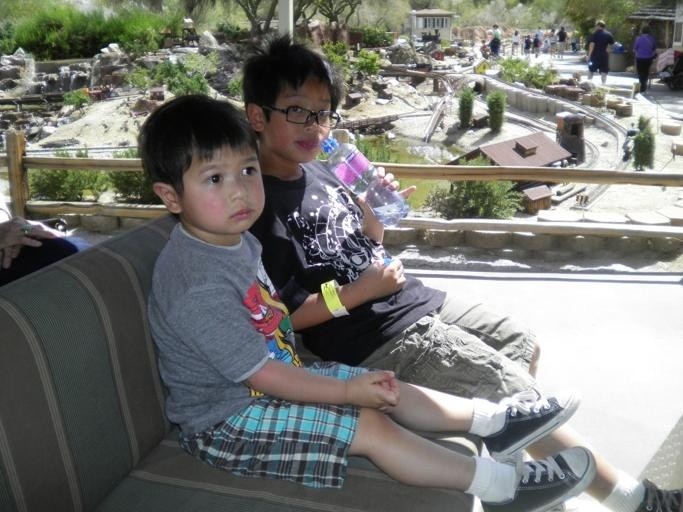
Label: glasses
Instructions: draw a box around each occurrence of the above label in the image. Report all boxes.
[259,104,341,130]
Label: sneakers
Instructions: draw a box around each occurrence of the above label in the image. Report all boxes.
[633,479,682,512]
[479,445,598,512]
[484,389,581,460]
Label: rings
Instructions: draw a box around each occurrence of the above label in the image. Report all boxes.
[21,222,30,235]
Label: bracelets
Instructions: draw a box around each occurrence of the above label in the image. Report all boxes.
[319,276,352,322]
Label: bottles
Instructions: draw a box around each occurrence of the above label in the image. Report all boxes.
[585,55,593,65]
[320,137,410,228]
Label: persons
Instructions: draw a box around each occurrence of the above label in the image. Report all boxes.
[631,23,655,95]
[477,23,580,63]
[583,20,621,84]
[0,188,79,305]
[238,35,682,512]
[138,92,601,512]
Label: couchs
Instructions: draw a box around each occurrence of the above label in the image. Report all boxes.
[1,213,486,512]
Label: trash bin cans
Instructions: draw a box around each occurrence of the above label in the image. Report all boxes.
[607,53,627,72]
[556,111,584,164]
